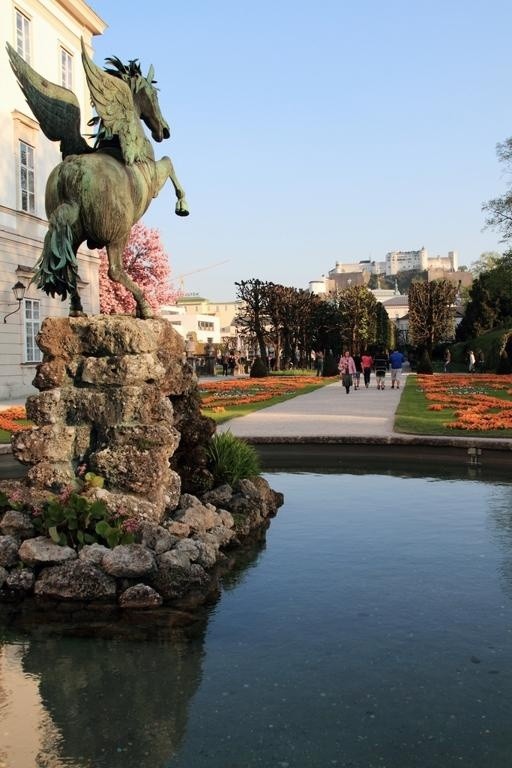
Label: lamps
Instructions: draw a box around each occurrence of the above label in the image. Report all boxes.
[4,281,26,324]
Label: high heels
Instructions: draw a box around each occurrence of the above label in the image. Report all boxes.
[365,382,370,388]
[346,389,349,393]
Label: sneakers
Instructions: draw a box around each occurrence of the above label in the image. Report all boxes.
[354,386,359,390]
[391,386,399,388]
[377,385,385,390]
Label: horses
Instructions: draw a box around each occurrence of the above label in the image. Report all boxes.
[4,33,191,320]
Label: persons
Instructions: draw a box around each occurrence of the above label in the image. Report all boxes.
[338,350,357,394]
[466,350,475,373]
[443,347,451,373]
[353,351,362,391]
[388,347,405,389]
[360,350,373,388]
[373,348,389,390]
[220,353,229,377]
[477,348,486,373]
